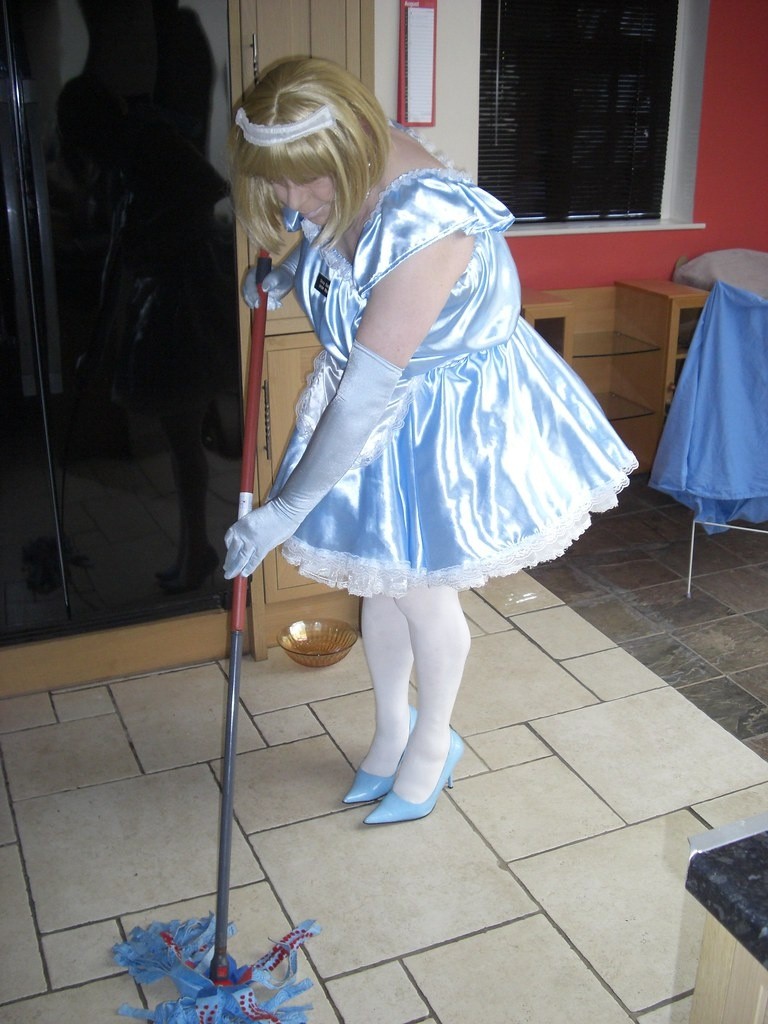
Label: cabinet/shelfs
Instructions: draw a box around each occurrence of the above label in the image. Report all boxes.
[221,0,376,661]
[518,279,712,481]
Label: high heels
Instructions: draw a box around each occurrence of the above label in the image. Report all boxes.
[341,704,418,804]
[363,727,463,824]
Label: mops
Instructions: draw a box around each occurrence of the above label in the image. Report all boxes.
[112,249,322,1024]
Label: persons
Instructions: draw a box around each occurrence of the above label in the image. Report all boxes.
[224,56,642,823]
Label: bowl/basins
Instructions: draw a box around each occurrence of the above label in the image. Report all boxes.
[276,616,358,668]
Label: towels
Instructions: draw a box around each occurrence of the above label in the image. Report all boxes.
[649,280,768,536]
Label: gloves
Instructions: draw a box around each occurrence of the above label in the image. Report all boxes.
[242,239,301,310]
[223,339,404,578]
